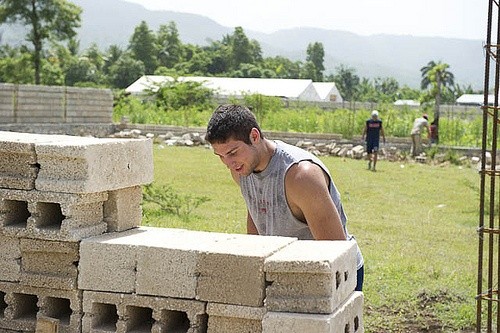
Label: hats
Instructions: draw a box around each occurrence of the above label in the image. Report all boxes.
[423,113,429,120]
[372,110,378,116]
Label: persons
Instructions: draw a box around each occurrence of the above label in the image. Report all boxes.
[363,111,386,171]
[204,103,365,292]
[410,114,442,158]
